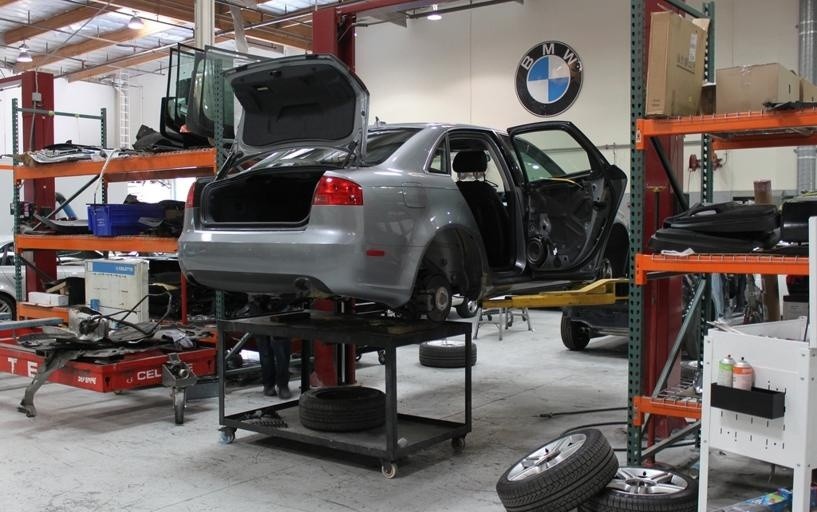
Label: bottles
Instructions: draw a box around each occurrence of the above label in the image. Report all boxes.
[719,354,735,388]
[732,356,753,390]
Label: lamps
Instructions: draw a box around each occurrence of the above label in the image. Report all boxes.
[425,2,442,21]
[125,10,143,28]
[12,37,34,63]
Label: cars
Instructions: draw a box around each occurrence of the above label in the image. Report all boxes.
[0,236,180,326]
[554,251,761,358]
[179,54,630,321]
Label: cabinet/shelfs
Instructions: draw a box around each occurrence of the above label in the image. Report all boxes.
[700,215,817,512]
[11,58,317,382]
[627,1,817,469]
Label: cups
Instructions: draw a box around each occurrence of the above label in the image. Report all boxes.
[90,299,99,312]
[752,180,771,205]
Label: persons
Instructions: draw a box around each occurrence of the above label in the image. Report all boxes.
[248,290,293,399]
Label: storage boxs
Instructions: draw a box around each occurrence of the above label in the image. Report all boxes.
[710,63,800,114]
[797,75,817,136]
[86,202,164,237]
[642,9,714,116]
